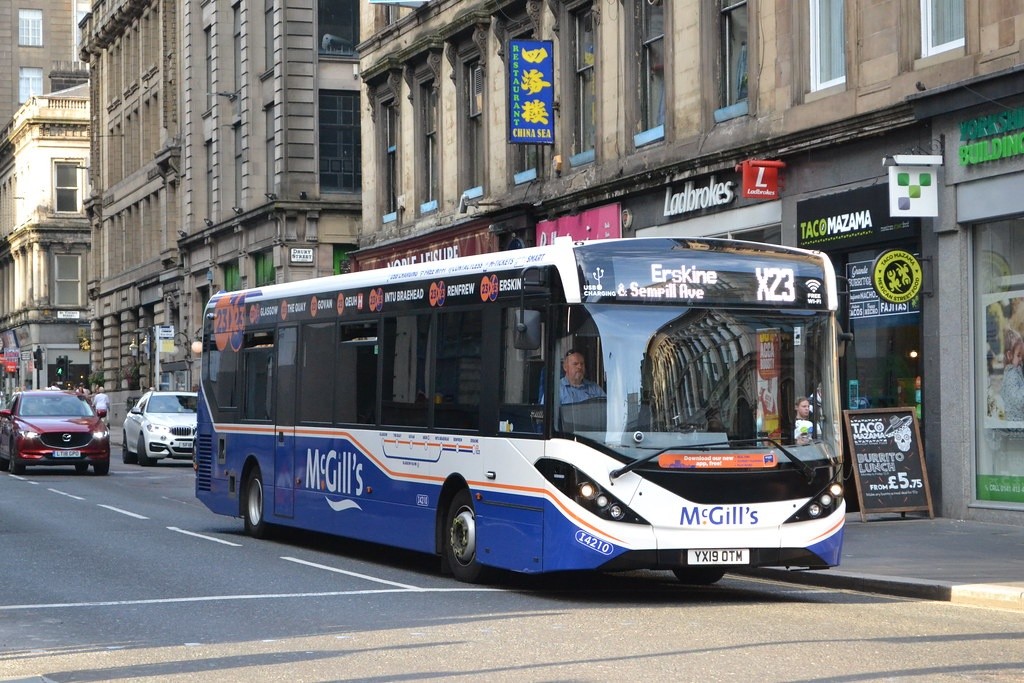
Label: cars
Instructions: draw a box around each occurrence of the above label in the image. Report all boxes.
[0,392,112,476]
[122,391,197,466]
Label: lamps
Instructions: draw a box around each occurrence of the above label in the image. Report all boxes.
[460,196,502,213]
[265,193,278,201]
[232,206,243,214]
[204,219,212,227]
[178,231,186,237]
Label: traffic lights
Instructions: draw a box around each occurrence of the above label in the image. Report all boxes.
[56,358,64,375]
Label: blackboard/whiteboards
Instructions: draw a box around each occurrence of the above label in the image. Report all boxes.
[844,408,934,512]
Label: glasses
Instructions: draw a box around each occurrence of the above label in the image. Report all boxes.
[566,349,581,358]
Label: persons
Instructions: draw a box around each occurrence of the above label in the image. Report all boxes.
[76,382,99,406]
[91,387,110,429]
[12,383,26,397]
[794,382,824,445]
[541,349,606,405]
[50,381,61,390]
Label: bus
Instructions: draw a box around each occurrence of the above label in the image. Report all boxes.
[195,236,855,583]
